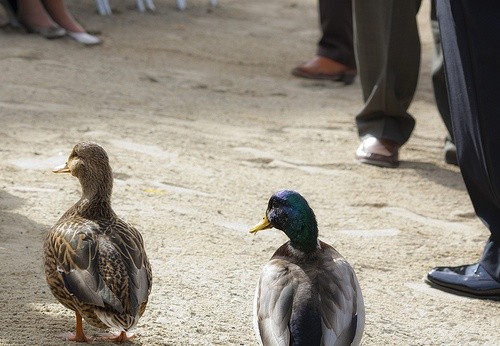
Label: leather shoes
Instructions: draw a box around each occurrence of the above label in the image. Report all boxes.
[424,262,500,301]
[356,137,399,168]
[292,55,358,85]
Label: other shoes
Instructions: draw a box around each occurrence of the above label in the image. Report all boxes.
[69,31,100,45]
[25,24,66,39]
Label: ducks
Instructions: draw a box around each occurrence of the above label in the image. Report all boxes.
[42,143,152,344]
[249,190,365,346]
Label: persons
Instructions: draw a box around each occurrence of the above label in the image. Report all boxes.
[18,0,105,45]
[424,0,500,301]
[291,0,359,85]
[355,0,459,168]
